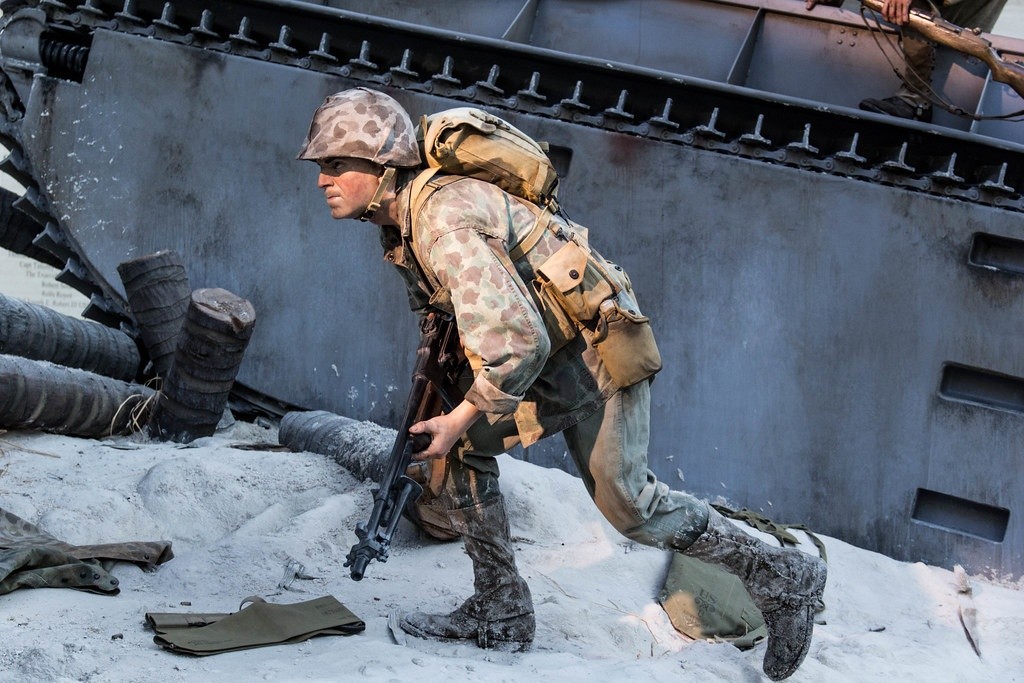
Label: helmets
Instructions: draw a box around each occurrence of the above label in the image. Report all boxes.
[293,87,421,167]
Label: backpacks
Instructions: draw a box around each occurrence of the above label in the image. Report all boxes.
[414,106,573,213]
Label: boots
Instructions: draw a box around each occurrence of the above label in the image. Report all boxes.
[681,500,829,682]
[400,495,536,653]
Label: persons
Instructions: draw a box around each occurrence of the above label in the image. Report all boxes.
[294,85,826,681]
[804,0,990,124]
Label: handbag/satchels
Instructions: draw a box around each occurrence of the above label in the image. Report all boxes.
[537,241,614,322]
[591,300,663,390]
[526,278,576,360]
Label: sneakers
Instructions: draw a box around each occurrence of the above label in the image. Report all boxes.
[858,95,933,124]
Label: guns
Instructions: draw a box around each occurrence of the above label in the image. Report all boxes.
[860,0,1024,100]
[342,311,466,582]
